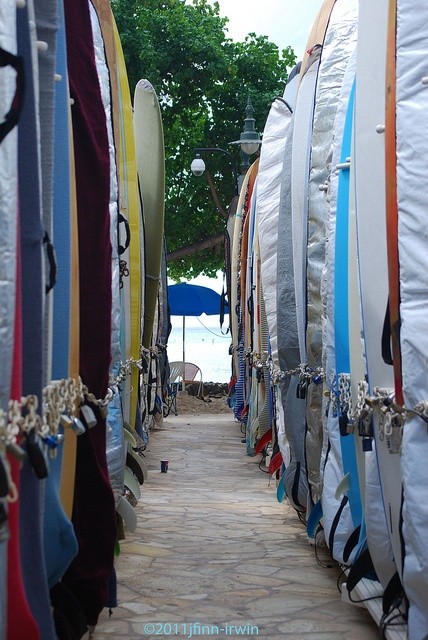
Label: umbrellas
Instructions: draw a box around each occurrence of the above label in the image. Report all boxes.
[167,282,230,391]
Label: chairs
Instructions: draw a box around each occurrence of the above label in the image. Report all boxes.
[177,362,204,398]
[168,361,184,383]
